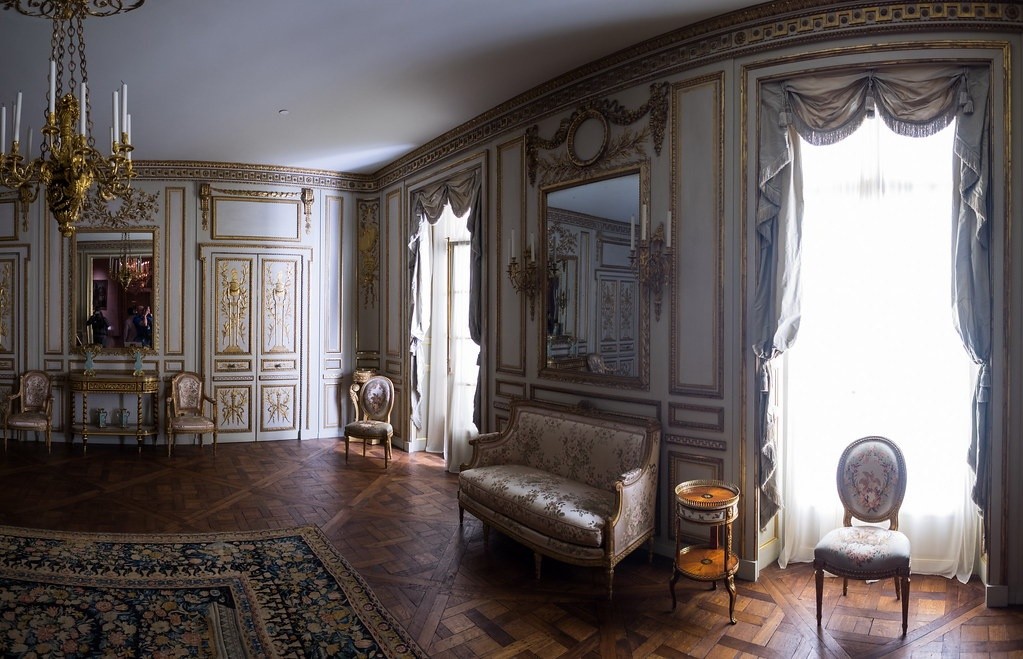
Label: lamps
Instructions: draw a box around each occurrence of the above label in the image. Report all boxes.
[110,232,151,291]
[535,159,652,395]
[1,1,145,237]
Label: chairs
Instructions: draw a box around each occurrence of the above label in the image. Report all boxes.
[2,369,55,456]
[812,436,911,642]
[166,371,218,458]
[342,376,395,468]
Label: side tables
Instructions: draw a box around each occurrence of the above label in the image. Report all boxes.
[68,372,161,457]
[667,479,739,628]
[349,368,381,445]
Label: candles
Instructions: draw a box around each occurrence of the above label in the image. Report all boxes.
[530,232,536,263]
[666,211,673,248]
[507,239,512,266]
[629,215,636,251]
[638,204,649,241]
[553,237,557,264]
[510,228,516,257]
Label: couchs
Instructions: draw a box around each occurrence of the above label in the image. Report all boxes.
[457,398,662,604]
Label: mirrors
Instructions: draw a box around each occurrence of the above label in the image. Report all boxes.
[69,226,160,357]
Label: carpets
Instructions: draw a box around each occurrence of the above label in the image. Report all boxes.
[0,524,434,659]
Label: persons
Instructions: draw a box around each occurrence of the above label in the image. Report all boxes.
[85,307,110,348]
[134,305,154,348]
